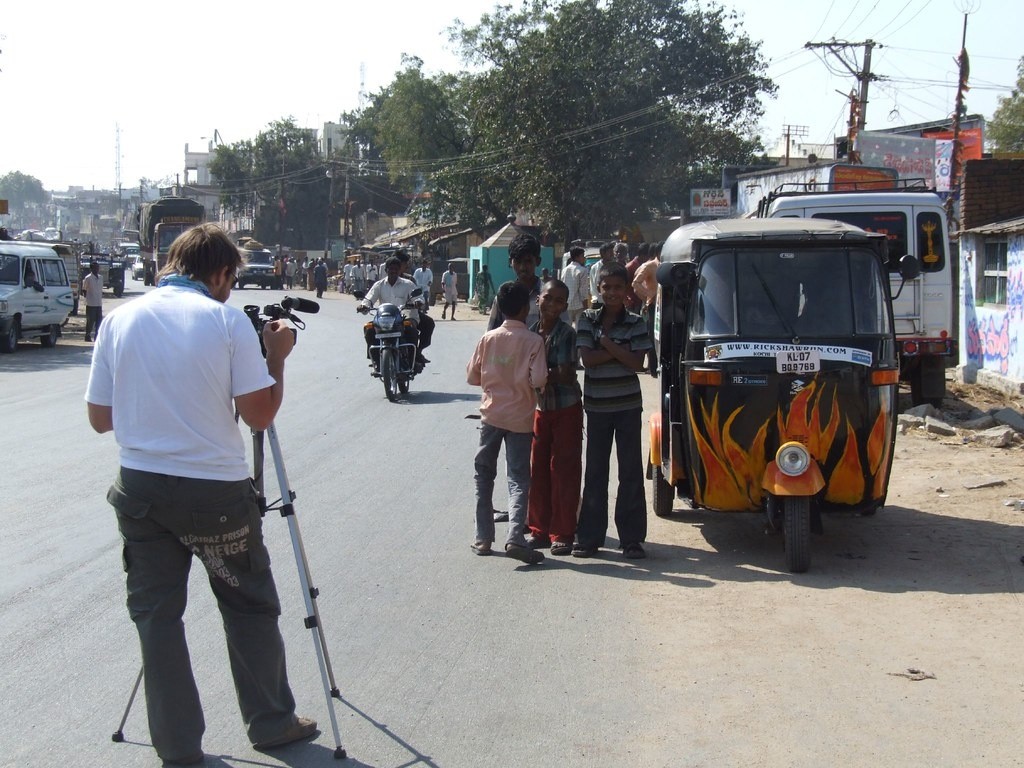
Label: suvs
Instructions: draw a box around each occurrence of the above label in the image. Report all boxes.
[0,244,74,352]
[119,242,142,268]
[742,181,959,412]
[235,247,276,291]
[133,256,145,282]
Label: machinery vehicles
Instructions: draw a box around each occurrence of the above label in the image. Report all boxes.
[81,240,126,299]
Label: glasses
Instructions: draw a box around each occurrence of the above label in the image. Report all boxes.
[228,269,238,289]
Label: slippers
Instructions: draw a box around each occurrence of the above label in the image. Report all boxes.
[505,543,544,564]
[470,541,493,555]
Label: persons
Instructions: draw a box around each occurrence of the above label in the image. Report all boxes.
[0,226,14,240]
[442,263,458,321]
[364,250,435,367]
[540,267,554,283]
[414,260,433,310]
[272,255,329,291]
[488,233,570,533]
[570,262,653,559]
[560,240,660,378]
[475,265,495,315]
[526,279,583,555]
[314,260,327,298]
[83,239,122,254]
[339,258,388,301]
[25,271,44,292]
[357,256,426,372]
[466,282,548,564]
[85,223,318,768]
[82,262,103,342]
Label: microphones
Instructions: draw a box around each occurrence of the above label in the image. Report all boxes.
[282,297,320,314]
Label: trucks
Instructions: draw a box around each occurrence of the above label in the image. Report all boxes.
[137,197,206,287]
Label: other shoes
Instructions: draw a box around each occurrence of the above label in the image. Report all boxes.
[570,543,598,556]
[258,716,317,748]
[85,338,91,342]
[161,748,205,768]
[525,536,551,548]
[551,539,573,555]
[416,355,431,363]
[524,523,531,534]
[369,364,373,367]
[376,367,380,372]
[623,541,644,558]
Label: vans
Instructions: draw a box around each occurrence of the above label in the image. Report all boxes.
[36,244,81,317]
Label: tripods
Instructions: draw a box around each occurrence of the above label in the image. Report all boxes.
[113,408,346,760]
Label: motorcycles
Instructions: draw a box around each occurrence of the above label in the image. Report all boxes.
[648,218,923,572]
[353,287,426,403]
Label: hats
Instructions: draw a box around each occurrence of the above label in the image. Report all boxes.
[393,251,409,261]
[384,258,401,273]
